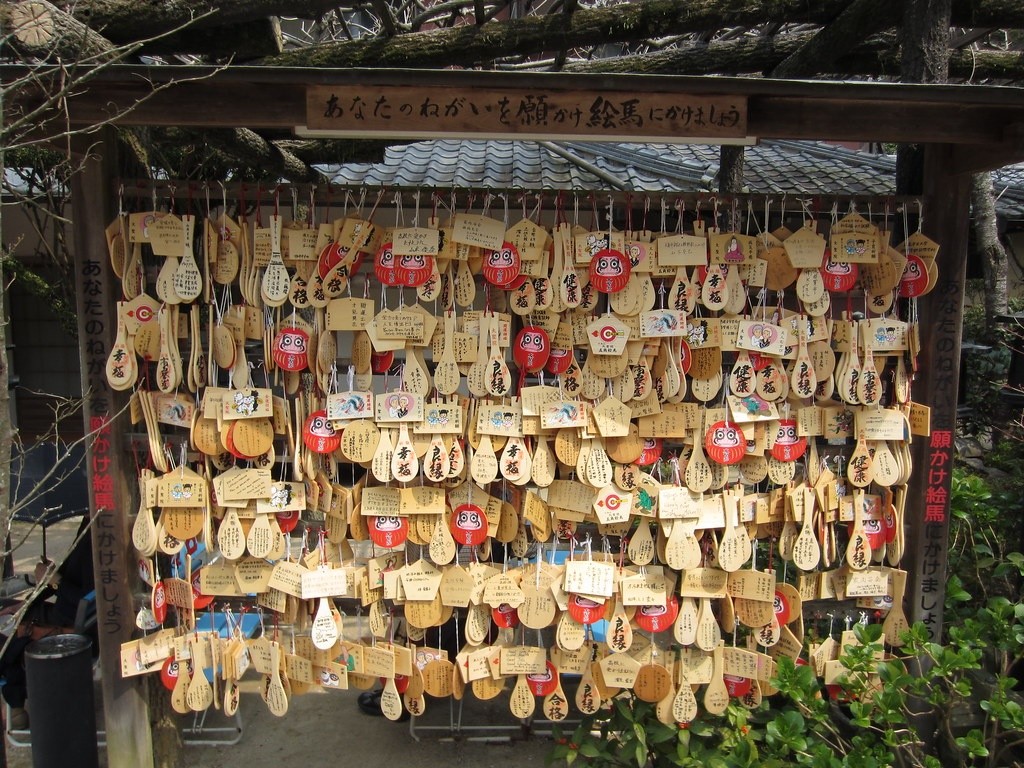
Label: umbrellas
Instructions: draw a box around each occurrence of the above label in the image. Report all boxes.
[9,445,89,586]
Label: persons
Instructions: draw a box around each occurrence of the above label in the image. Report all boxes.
[0,514,95,730]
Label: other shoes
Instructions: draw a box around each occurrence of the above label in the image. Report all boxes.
[1,710,30,731]
[355,687,411,724]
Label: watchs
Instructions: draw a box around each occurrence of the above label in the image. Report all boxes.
[52,578,62,592]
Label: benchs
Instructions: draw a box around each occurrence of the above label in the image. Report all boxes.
[6,532,625,747]
